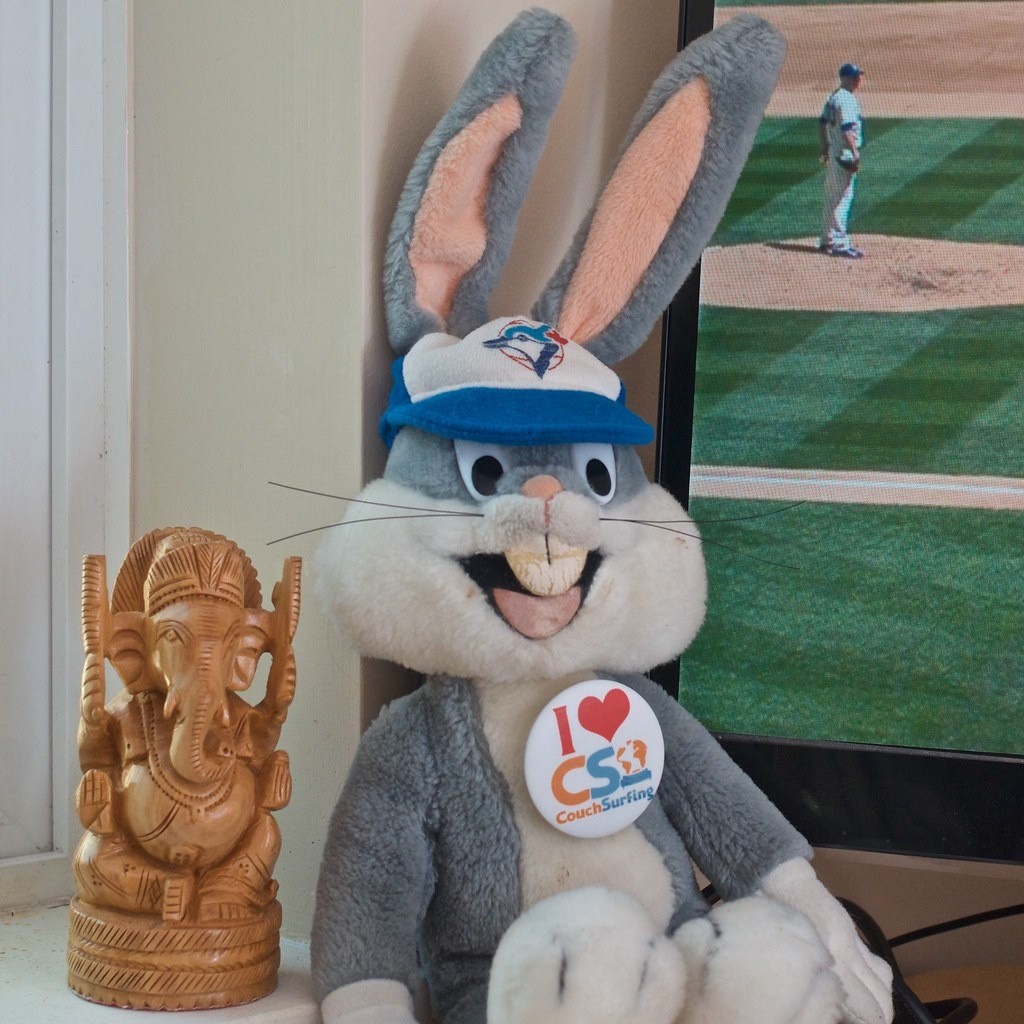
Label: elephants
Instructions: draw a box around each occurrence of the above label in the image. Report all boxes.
[81,527,302,919]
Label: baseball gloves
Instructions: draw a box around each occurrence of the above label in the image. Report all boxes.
[835,156,861,174]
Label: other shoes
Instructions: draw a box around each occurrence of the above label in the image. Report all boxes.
[829,247,863,258]
[820,244,831,253]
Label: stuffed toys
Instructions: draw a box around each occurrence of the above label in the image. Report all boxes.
[312,6,894,1024]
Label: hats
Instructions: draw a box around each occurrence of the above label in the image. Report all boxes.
[839,63,864,76]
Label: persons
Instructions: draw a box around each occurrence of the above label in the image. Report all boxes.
[818,64,863,258]
